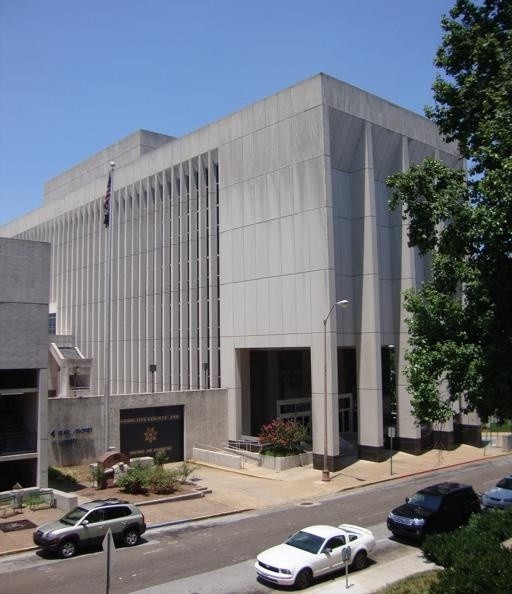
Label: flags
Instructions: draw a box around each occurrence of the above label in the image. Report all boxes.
[103,170,112,228]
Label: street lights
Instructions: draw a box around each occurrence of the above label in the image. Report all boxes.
[323,299,351,481]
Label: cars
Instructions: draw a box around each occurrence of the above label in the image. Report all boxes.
[482,475,512,510]
[255,523,377,590]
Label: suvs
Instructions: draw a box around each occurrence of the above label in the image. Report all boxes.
[387,482,481,544]
[34,497,146,558]
